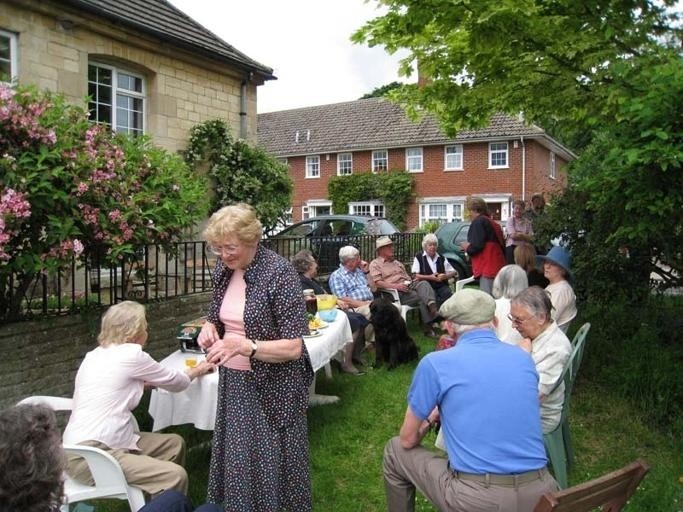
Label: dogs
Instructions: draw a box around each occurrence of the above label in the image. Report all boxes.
[369,297,420,372]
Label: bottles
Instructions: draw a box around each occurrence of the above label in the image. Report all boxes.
[304,289,318,317]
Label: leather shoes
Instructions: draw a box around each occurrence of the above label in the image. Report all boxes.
[426,329,439,338]
[338,366,367,377]
[427,304,438,316]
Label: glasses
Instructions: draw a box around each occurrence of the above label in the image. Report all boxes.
[210,245,238,257]
[506,314,534,324]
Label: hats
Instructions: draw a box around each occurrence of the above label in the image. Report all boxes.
[374,236,392,250]
[439,288,496,325]
[534,247,574,282]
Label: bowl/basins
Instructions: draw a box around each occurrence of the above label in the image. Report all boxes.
[315,294,337,312]
[318,309,337,322]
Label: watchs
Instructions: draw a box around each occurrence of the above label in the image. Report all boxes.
[426,417,432,435]
[248,338,258,361]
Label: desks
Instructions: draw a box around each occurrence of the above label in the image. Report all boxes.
[148,309,354,433]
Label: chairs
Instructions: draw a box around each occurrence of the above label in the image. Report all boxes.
[545,321,592,488]
[555,309,578,333]
[379,288,419,324]
[15,395,146,512]
[533,458,652,512]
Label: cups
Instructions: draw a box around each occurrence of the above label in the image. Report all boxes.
[182,353,199,370]
[305,295,318,319]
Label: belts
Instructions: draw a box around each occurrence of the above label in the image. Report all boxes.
[449,463,550,486]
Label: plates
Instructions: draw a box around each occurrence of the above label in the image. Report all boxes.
[301,328,322,338]
[308,319,328,329]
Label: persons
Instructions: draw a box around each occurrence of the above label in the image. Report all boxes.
[383,289,562,512]
[197,204,315,512]
[409,233,459,334]
[457,197,507,297]
[1,403,225,511]
[506,200,535,268]
[524,195,552,251]
[488,266,532,353]
[532,249,577,330]
[331,245,401,353]
[289,250,362,375]
[511,243,544,285]
[61,302,215,511]
[370,236,442,342]
[507,285,572,436]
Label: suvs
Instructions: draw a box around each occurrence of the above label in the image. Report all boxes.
[266,216,400,271]
[435,222,508,279]
